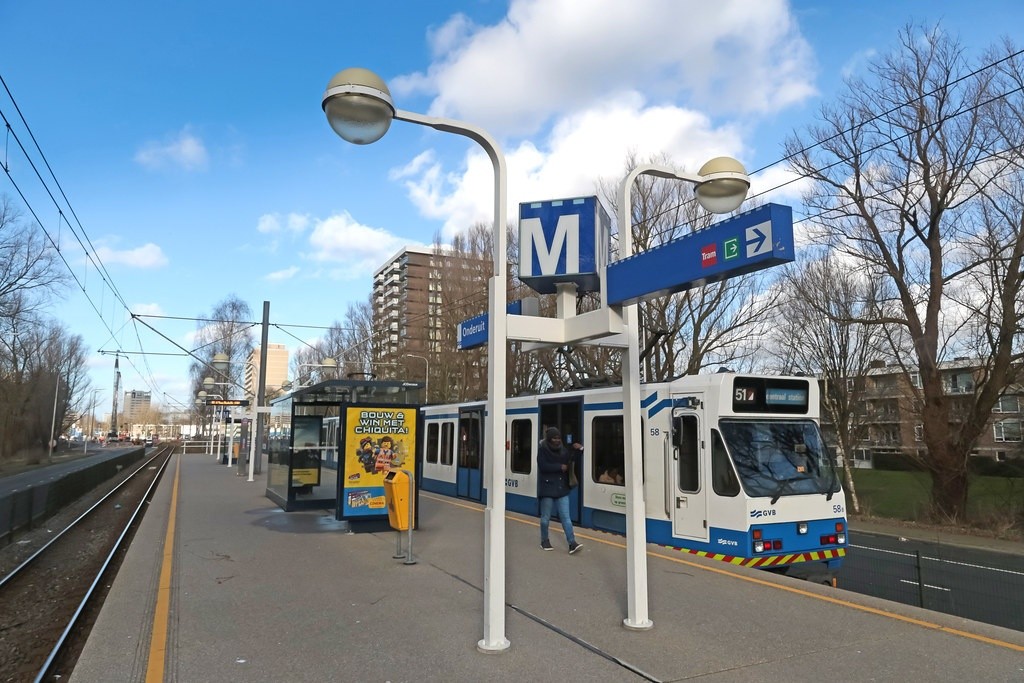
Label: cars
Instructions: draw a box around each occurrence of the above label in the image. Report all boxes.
[59,433,128,443]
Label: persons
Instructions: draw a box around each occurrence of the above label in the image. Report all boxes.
[537,427,583,554]
[598,464,623,484]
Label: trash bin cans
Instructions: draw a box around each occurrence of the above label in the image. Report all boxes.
[384,470,416,530]
[234,443,239,458]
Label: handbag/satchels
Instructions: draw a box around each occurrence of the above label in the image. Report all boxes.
[569,462,578,488]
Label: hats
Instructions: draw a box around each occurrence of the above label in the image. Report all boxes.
[545,427,561,442]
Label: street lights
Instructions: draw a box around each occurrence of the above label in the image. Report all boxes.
[194,353,261,482]
[616,157,753,631]
[49,370,83,458]
[405,353,430,404]
[319,68,513,650]
[85,388,110,454]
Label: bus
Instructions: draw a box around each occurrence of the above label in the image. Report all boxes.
[320,371,851,583]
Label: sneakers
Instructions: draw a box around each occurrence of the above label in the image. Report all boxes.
[569,542,583,554]
[540,539,554,550]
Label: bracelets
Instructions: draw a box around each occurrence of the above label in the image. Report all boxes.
[579,447,584,450]
